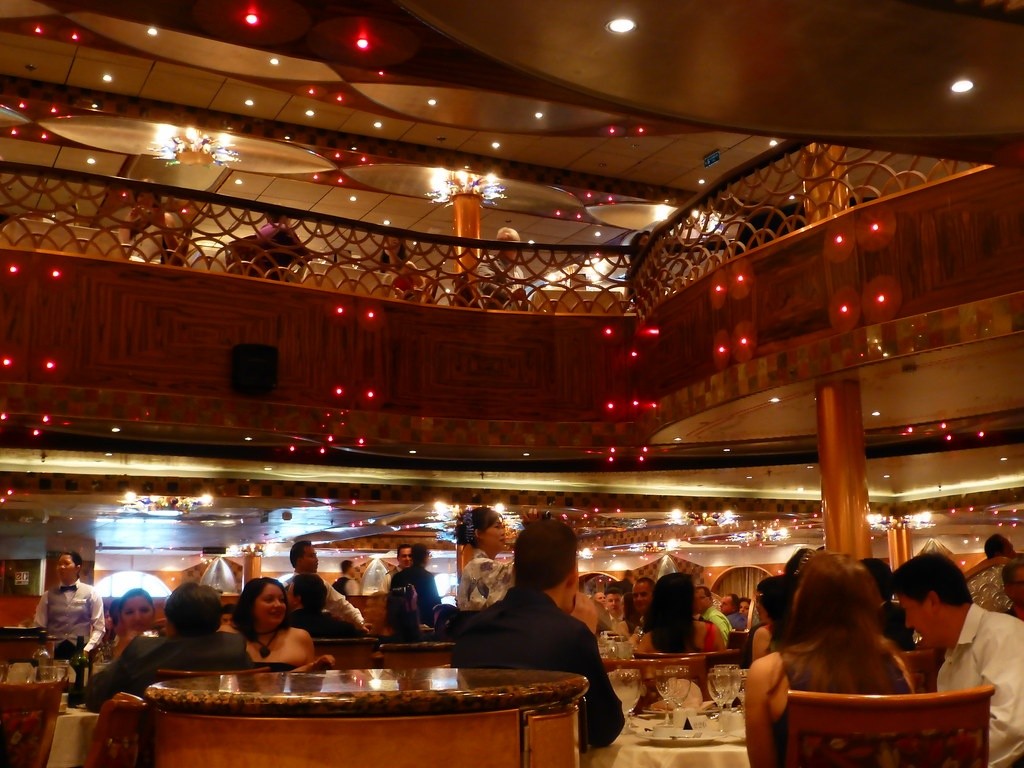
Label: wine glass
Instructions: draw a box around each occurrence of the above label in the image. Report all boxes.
[607,663,749,734]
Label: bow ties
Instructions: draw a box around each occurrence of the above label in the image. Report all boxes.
[59,585,77,593]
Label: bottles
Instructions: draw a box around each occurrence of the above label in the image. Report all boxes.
[67,635,90,707]
[30,630,54,670]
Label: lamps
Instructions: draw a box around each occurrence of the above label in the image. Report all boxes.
[425,168,507,210]
[145,128,243,169]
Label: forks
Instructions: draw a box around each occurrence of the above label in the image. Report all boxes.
[669,731,703,738]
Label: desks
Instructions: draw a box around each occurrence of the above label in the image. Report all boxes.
[1,219,122,260]
[579,716,752,768]
[302,261,393,298]
[531,288,626,312]
[144,669,589,767]
[45,708,98,768]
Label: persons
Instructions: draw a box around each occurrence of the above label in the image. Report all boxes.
[0,505,1024,768]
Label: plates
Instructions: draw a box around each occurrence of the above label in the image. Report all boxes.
[635,730,728,748]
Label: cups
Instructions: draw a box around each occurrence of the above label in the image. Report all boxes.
[35,665,57,686]
[6,662,35,684]
[597,630,634,660]
[58,693,70,715]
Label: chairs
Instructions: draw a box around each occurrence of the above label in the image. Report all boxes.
[600,631,996,768]
[0,636,455,768]
[452,271,477,307]
[227,234,260,279]
